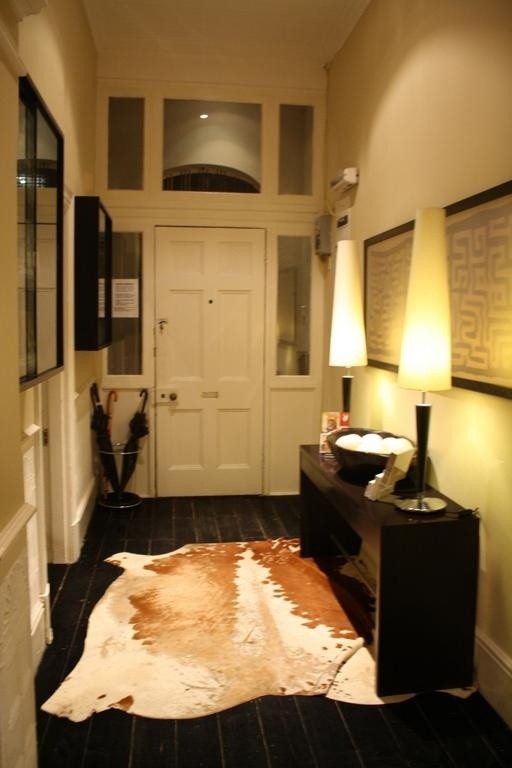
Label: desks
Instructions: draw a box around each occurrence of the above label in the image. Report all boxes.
[297,444,480,697]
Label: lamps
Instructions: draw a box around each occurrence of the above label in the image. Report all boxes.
[391,207,453,513]
[327,239,367,425]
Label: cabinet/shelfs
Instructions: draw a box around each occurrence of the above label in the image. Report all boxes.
[75,195,114,350]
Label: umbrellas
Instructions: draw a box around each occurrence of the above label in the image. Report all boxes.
[119,388,150,495]
[100,389,118,498]
[91,383,122,504]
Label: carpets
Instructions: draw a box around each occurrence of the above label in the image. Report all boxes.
[41,536,475,721]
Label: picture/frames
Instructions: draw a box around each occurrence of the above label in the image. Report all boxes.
[362,179,512,400]
